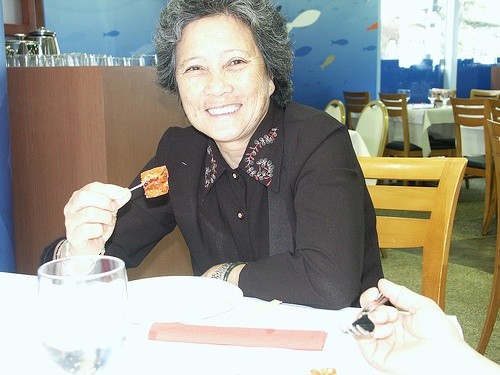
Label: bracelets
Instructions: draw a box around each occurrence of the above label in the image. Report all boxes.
[212,263,232,279]
[223,262,246,281]
[55,238,96,276]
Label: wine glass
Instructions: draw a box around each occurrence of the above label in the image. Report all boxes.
[439,90,450,106]
[427,88,437,105]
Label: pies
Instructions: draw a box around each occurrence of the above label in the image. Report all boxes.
[140,165,169,198]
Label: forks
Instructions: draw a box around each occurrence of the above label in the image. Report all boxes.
[339,292,388,335]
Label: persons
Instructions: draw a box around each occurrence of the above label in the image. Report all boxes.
[39,0,384,310]
[354,278,500,375]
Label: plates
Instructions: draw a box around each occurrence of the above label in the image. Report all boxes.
[123,275,244,340]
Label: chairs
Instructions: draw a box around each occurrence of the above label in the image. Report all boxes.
[325,87,500,355]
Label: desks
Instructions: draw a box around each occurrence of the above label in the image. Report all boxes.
[0,272,464,375]
[351,104,462,159]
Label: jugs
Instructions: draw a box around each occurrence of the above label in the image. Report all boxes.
[28,26,60,54]
[5,33,34,54]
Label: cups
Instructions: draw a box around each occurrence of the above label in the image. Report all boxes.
[398,89,411,101]
[33,254,130,374]
[5,52,157,67]
